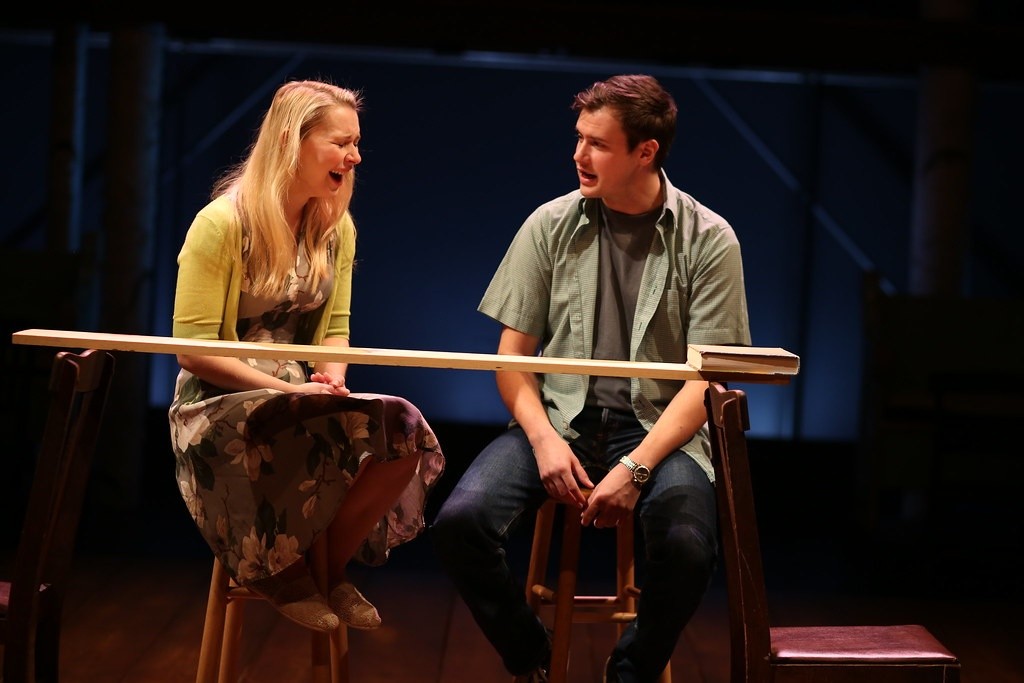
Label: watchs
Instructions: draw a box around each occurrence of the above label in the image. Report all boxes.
[618,454,651,484]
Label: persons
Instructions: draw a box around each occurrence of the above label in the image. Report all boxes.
[428,74,754,681]
[168,80,428,630]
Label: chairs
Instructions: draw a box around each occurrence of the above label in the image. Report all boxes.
[0,345,121,683]
[701,377,960,683]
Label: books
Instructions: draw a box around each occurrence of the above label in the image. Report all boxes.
[686,343,800,376]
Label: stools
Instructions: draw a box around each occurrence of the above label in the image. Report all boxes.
[507,481,673,682]
[196,506,354,683]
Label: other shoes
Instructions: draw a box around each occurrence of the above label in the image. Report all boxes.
[322,572,383,633]
[243,564,338,635]
